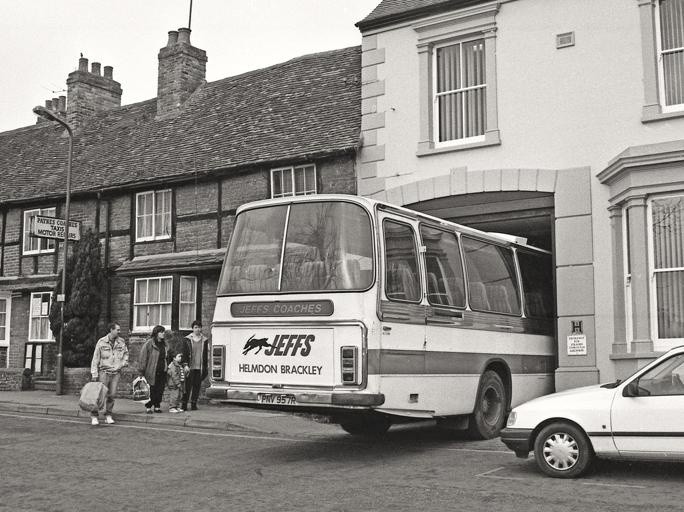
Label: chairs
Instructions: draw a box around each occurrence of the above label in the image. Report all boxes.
[230,259,550,319]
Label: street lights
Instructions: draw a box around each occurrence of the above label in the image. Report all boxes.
[31,103,76,398]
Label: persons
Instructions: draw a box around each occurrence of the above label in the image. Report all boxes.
[165,350,191,414]
[138,324,173,415]
[90,321,130,427]
[176,319,208,412]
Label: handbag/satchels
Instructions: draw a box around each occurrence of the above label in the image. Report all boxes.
[79,381,109,412]
[132,376,150,402]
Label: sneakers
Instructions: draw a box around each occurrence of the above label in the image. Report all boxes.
[183,403,198,410]
[169,408,184,412]
[105,415,115,424]
[90,415,99,425]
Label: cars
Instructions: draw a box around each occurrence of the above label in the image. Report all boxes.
[498,344,684,480]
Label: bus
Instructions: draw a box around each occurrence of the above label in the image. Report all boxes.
[206,195,556,441]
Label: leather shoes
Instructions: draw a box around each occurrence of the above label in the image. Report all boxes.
[146,408,162,414]
